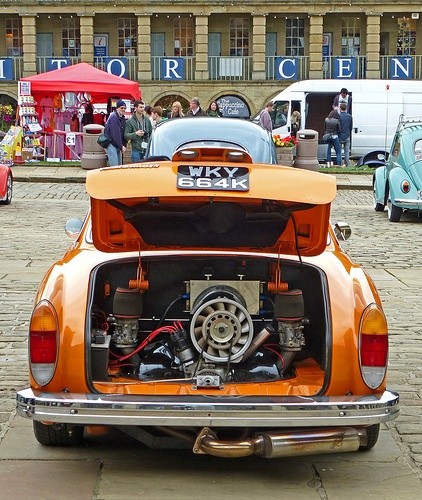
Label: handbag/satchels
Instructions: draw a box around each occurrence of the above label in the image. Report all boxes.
[97,134,112,149]
[323,134,330,143]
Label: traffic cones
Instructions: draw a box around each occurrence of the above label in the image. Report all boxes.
[12,141,25,163]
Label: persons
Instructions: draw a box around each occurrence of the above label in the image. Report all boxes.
[324,110,342,167]
[124,101,152,163]
[184,99,209,117]
[332,88,352,114]
[206,101,223,117]
[102,100,127,166]
[167,101,185,119]
[338,103,352,167]
[276,104,298,127]
[144,106,156,125]
[260,102,275,133]
[150,106,168,125]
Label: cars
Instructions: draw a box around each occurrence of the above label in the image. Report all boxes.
[15,114,401,458]
[372,115,421,222]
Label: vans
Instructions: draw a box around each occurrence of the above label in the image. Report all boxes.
[250,78,422,163]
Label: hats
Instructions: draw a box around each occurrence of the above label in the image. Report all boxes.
[117,101,126,107]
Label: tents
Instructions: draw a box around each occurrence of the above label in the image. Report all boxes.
[20,62,141,132]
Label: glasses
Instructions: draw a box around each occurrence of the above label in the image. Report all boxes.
[121,107,126,109]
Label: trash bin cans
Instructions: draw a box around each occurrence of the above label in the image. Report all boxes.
[81,123,106,168]
[295,130,322,170]
